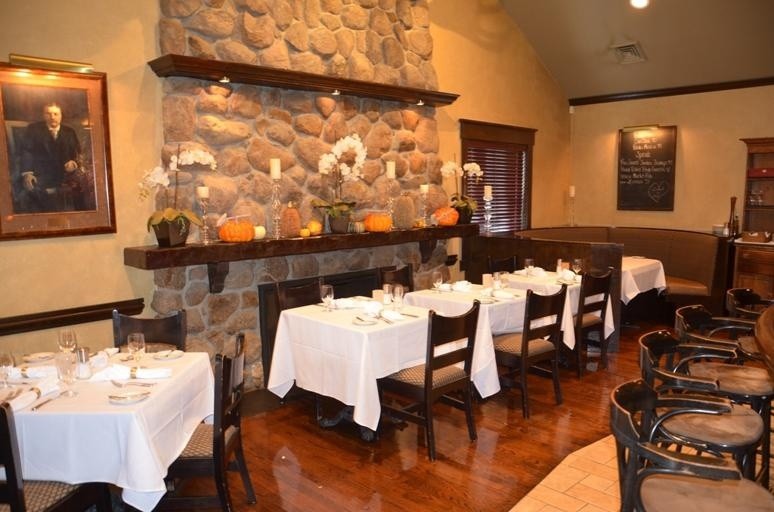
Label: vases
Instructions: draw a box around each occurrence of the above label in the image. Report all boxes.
[149,215,191,247]
[325,209,351,234]
[449,203,473,224]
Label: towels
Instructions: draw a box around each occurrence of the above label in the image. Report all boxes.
[331,296,360,311]
[4,384,59,414]
[362,300,404,325]
[107,361,173,380]
[2,365,58,379]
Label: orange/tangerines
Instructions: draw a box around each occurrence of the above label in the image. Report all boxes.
[307,220,322,235]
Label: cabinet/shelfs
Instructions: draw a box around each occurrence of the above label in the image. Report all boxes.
[733,135,772,315]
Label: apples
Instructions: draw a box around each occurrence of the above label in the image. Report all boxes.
[300,229,310,236]
[253,226,265,240]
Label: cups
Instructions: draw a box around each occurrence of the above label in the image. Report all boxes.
[382,283,404,311]
[498,270,510,288]
[482,274,491,288]
[711,224,723,232]
[127,332,147,360]
[57,328,77,352]
[525,258,535,278]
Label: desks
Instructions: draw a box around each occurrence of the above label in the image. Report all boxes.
[402,252,668,374]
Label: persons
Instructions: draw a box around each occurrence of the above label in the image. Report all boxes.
[14,99,97,214]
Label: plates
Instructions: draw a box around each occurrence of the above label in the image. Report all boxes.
[353,316,378,325]
[110,390,151,406]
[154,349,185,360]
[24,351,55,363]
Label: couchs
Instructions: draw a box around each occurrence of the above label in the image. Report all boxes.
[511,224,730,330]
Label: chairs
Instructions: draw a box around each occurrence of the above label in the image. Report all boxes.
[109,304,186,352]
[370,297,482,463]
[273,274,328,326]
[602,284,774,511]
[557,263,617,380]
[374,259,415,296]
[115,332,256,511]
[0,399,114,512]
[488,283,568,420]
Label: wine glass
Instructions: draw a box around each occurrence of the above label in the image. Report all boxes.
[319,284,335,312]
[572,258,583,274]
[56,353,82,400]
[431,271,443,293]
[1,350,14,391]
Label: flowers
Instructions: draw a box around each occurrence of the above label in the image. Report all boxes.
[305,133,370,219]
[436,160,485,212]
[131,140,224,233]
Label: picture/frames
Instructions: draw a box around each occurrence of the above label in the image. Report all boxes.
[0,61,117,241]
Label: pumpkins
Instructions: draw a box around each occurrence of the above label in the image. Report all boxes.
[218,220,254,242]
[280,208,301,238]
[435,208,459,225]
[393,196,417,227]
[363,213,393,232]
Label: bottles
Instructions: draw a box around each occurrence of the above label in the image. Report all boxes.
[733,214,741,236]
[723,222,729,235]
[750,189,764,207]
[77,346,91,380]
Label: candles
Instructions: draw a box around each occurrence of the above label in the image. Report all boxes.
[194,185,209,201]
[483,184,493,200]
[385,160,396,178]
[419,184,430,194]
[268,158,280,180]
[568,185,575,198]
